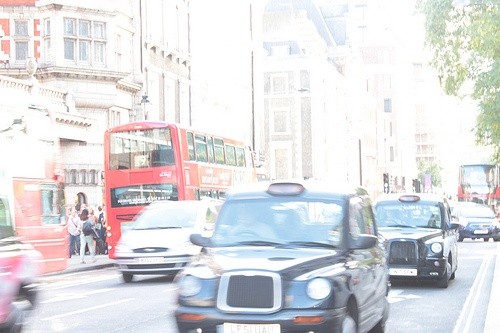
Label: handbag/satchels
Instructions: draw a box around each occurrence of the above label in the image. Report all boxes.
[82,220,93,236]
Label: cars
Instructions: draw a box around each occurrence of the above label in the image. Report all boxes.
[371,192,461,289]
[0,233,46,333]
[450,201,500,243]
[113,198,225,283]
[170,180,393,333]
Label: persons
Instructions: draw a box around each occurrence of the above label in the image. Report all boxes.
[225,202,275,239]
[77,208,99,263]
[66,201,108,258]
[381,209,401,224]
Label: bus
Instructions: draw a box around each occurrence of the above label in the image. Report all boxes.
[103,120,259,260]
[455,163,500,219]
[0,171,73,277]
